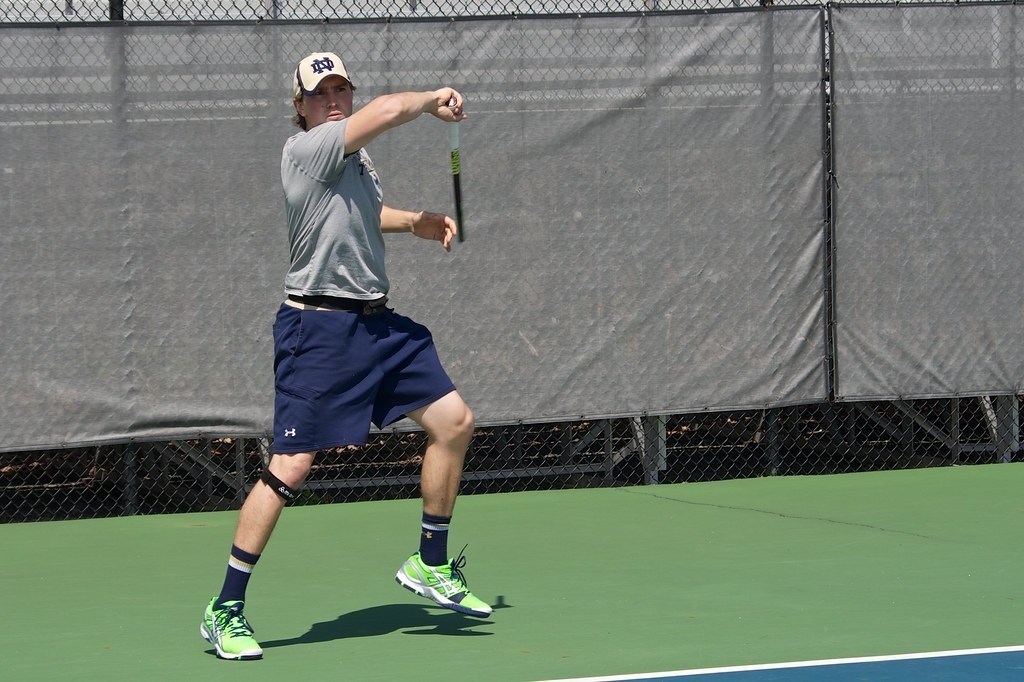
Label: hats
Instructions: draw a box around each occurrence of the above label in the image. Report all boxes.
[292,51,353,99]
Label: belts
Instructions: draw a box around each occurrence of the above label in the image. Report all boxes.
[287,289,390,312]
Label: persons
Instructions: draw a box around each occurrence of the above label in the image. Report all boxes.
[200,51,493,660]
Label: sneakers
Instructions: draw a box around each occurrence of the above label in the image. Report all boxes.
[394,551,493,620]
[199,594,264,662]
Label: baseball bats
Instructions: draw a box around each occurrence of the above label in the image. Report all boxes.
[447,99,466,244]
